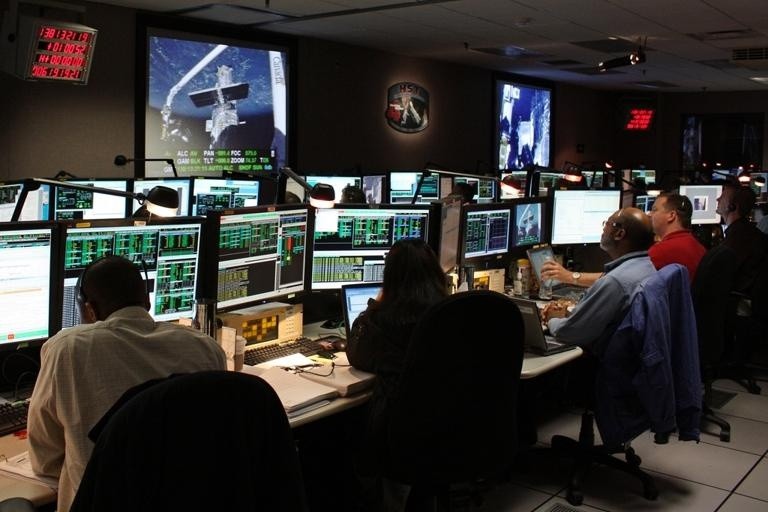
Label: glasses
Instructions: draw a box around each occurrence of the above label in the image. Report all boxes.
[601,220,622,229]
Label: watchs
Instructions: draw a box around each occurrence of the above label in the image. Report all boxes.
[572,272,581,285]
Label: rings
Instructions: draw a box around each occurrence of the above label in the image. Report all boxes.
[550,271,552,274]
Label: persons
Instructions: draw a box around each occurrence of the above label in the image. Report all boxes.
[514,207,655,446]
[448,182,478,203]
[631,178,649,207]
[345,240,453,441]
[700,185,768,291]
[26,253,229,511]
[538,192,707,287]
[341,186,366,203]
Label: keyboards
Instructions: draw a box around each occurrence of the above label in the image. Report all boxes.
[0,400,30,436]
[237,336,325,367]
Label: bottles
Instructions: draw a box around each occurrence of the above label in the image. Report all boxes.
[513,257,530,296]
[539,277,553,300]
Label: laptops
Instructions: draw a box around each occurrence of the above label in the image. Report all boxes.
[527,245,589,300]
[512,297,578,356]
[470,268,509,297]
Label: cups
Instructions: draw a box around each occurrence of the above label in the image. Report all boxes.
[234,335,248,371]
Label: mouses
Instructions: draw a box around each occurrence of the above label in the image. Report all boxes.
[333,339,348,351]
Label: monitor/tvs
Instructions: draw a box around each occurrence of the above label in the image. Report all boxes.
[308,206,432,295]
[505,196,547,257]
[546,184,625,246]
[635,193,659,216]
[53,177,132,221]
[453,169,662,206]
[342,282,387,344]
[205,203,314,313]
[132,25,293,179]
[390,171,441,206]
[129,177,194,220]
[0,220,59,352]
[285,176,389,208]
[711,169,768,240]
[54,215,207,331]
[678,183,723,225]
[460,205,514,268]
[490,72,555,173]
[189,175,264,219]
[1,177,55,223]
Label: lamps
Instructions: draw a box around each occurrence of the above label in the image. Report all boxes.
[706,168,765,189]
[410,168,521,204]
[115,156,176,176]
[273,166,334,209]
[11,176,179,223]
[583,159,664,198]
[525,165,582,197]
